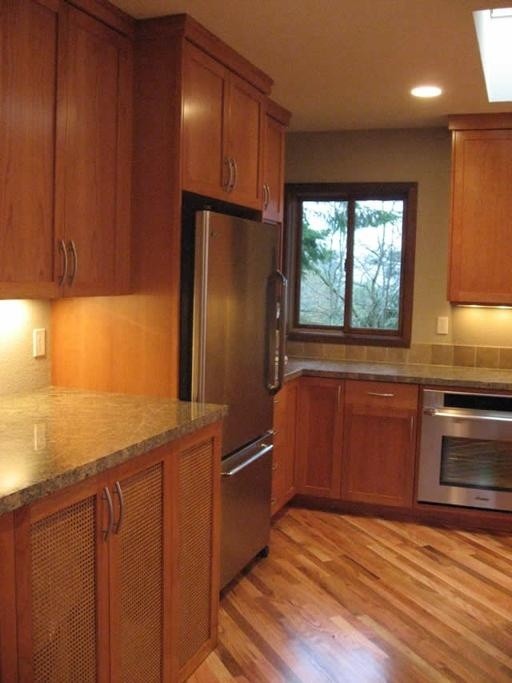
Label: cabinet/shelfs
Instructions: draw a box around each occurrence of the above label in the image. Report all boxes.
[0,0,133,300]
[446,112,512,306]
[134,15,273,211]
[16,421,222,683]
[341,379,423,519]
[296,376,343,511]
[261,97,292,223]
[270,377,296,516]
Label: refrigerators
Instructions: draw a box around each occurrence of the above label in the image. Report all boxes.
[178,208,287,598]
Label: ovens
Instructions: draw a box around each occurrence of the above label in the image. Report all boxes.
[418,388,512,513]
